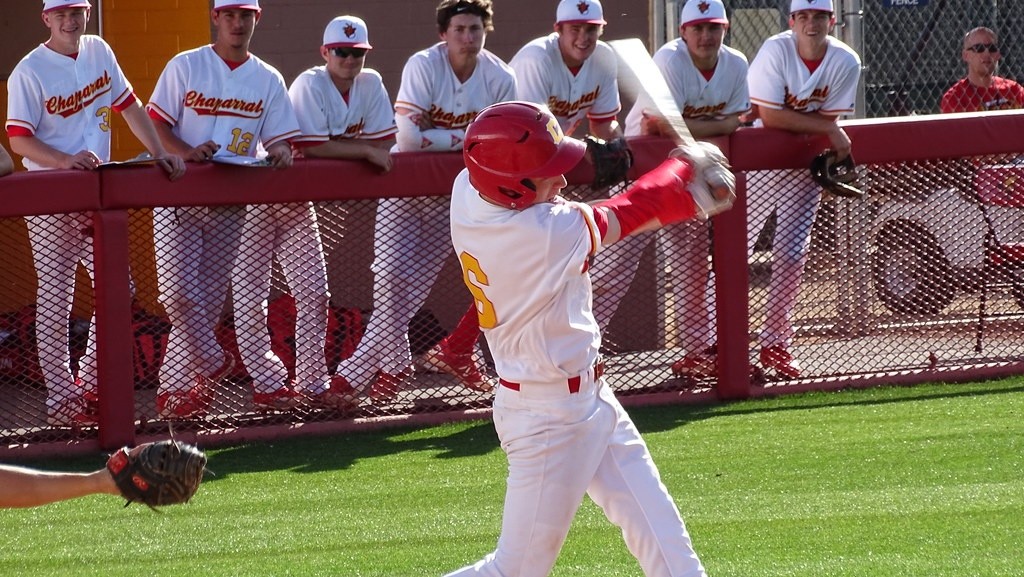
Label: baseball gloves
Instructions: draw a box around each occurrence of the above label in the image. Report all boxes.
[105,439,208,507]
[809,148,864,199]
[584,132,634,191]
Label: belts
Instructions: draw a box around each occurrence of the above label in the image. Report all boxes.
[500,355,604,393]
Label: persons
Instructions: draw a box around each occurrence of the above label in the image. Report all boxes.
[5,0,186,427]
[0,439,207,508]
[425,0,623,392]
[442,102,735,577]
[329,0,519,406]
[231,16,399,410]
[146,0,302,422]
[707,0,860,377]
[941,28,1024,167]
[0,142,14,177]
[586,0,752,380]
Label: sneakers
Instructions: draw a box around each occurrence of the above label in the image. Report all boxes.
[73,375,99,401]
[419,339,493,392]
[46,397,99,426]
[295,386,358,409]
[330,376,359,401]
[254,385,309,412]
[366,364,415,398]
[157,391,208,420]
[190,350,236,418]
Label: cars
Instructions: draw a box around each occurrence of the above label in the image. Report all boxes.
[870,181,1024,316]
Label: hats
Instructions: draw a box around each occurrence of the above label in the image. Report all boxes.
[682,0,729,26]
[789,0,834,14]
[214,0,261,11]
[556,0,607,27]
[323,15,373,50]
[43,0,91,12]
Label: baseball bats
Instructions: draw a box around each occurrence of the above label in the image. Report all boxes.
[595,38,729,202]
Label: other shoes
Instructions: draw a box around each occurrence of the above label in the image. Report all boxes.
[761,345,801,379]
[684,357,719,376]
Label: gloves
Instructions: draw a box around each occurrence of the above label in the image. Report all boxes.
[686,164,736,220]
[668,141,731,179]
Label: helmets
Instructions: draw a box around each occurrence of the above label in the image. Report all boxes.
[463,101,587,211]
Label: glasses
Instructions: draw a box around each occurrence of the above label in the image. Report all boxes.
[966,44,998,53]
[329,47,369,58]
